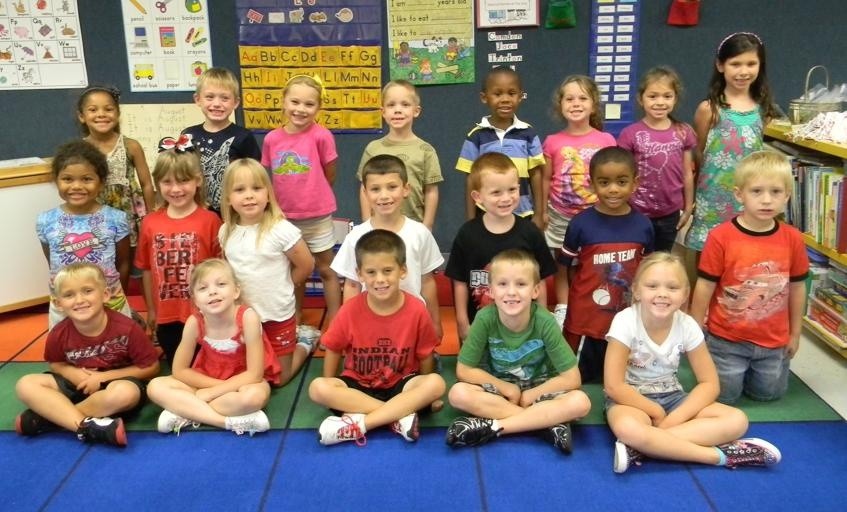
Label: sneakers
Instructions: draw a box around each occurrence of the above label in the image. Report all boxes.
[297,325,321,351]
[74,415,127,447]
[717,437,782,470]
[230,409,270,437]
[317,412,420,447]
[16,408,54,436]
[158,410,201,437]
[446,416,504,451]
[548,423,572,454]
[613,438,645,473]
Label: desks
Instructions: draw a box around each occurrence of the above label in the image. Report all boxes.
[0,157,56,314]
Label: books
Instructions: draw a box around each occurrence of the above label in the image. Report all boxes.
[761,138,847,343]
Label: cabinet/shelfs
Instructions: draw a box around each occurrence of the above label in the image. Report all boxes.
[762,118,847,361]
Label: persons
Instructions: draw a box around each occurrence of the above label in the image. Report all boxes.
[217,156,324,388]
[146,256,284,438]
[132,148,226,372]
[445,152,560,350]
[603,249,784,475]
[13,261,161,449]
[328,152,446,350]
[309,229,447,447]
[690,149,811,406]
[445,247,592,456]
[33,138,132,334]
[76,28,782,328]
[558,144,658,385]
